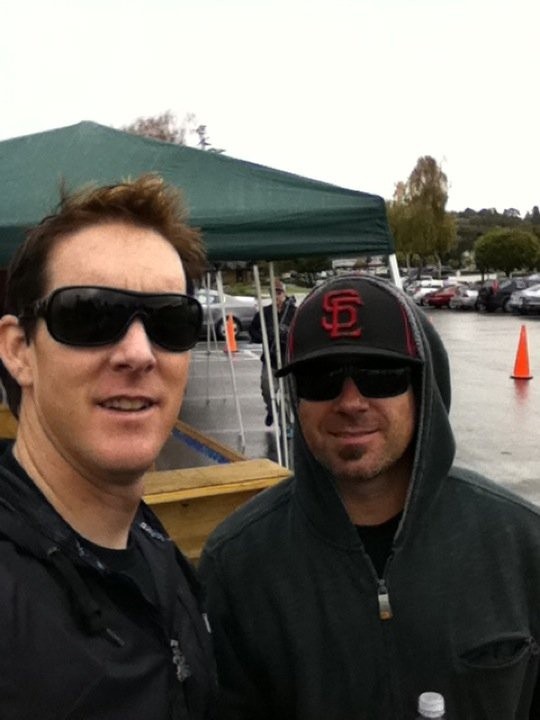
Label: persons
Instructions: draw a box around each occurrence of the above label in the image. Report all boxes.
[2,172,218,720]
[198,272,538,719]
[249,280,297,438]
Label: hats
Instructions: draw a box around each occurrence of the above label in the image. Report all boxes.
[272,279,427,367]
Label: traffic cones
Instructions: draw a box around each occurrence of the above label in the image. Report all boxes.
[506,324,533,380]
[223,311,239,352]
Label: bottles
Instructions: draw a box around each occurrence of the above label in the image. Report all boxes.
[417,692,447,720]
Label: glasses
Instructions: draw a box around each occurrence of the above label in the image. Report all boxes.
[289,359,416,403]
[16,286,206,354]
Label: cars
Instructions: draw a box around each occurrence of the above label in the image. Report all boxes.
[399,264,540,316]
[194,287,260,342]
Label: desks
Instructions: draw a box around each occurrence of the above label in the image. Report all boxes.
[144,457,295,571]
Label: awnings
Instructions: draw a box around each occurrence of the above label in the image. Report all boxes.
[0,121,403,470]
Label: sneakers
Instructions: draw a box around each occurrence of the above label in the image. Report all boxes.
[284,423,294,439]
[264,411,273,427]
